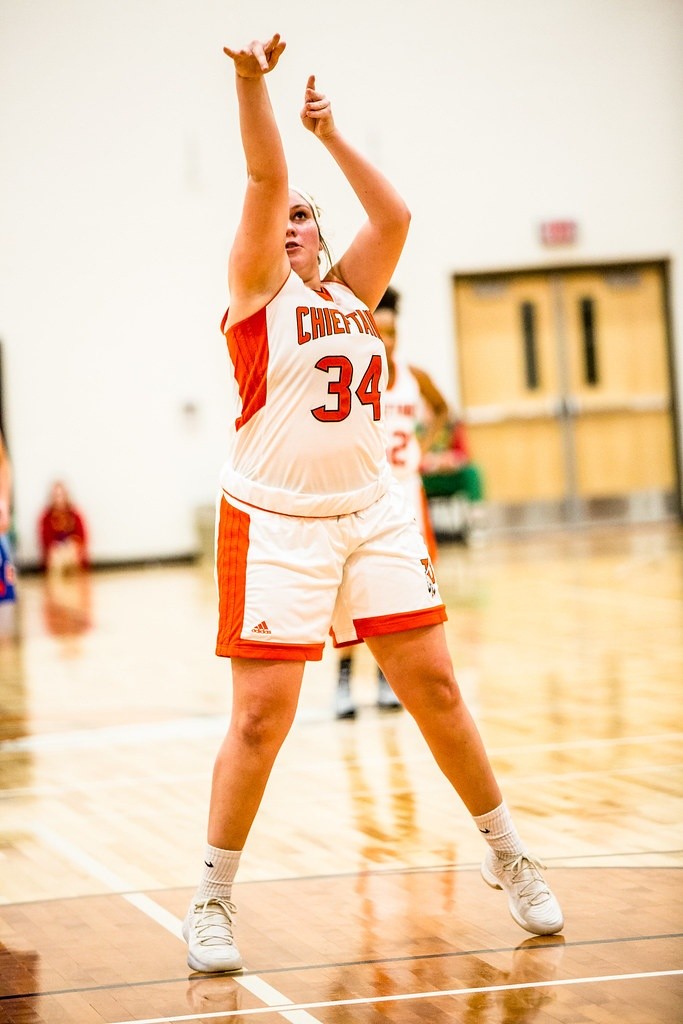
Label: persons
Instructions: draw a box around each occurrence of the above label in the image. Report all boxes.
[181,34,564,975]
[335,285,449,717]
[36,483,92,577]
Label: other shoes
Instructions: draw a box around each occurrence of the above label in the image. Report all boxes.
[333,673,356,717]
[374,679,401,706]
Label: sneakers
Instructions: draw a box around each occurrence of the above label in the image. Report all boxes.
[476,846,566,935]
[180,895,241,971]
